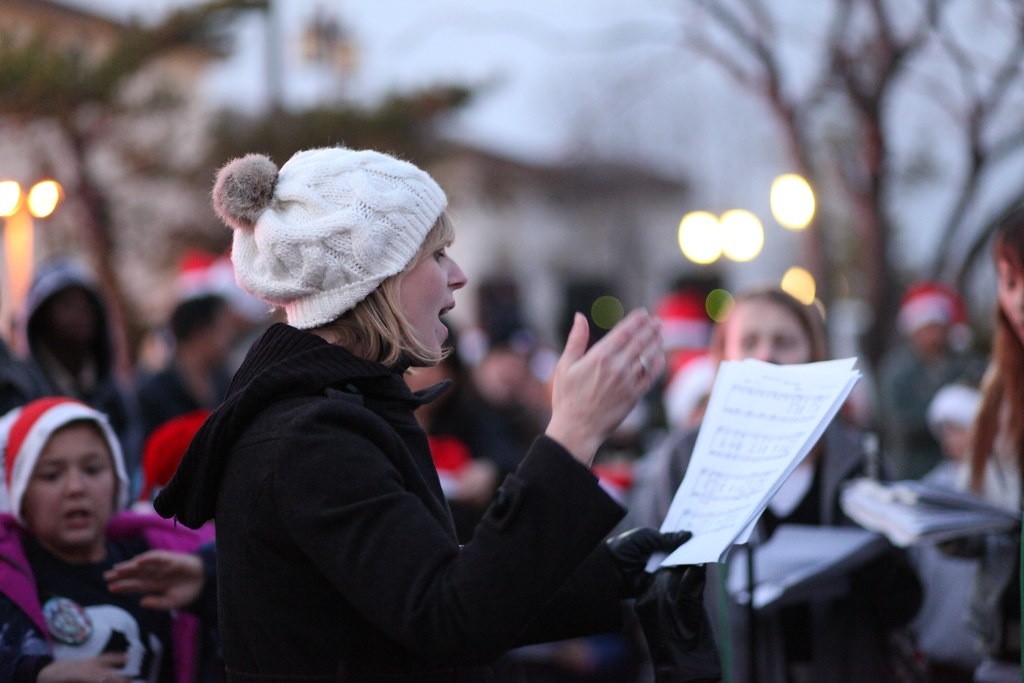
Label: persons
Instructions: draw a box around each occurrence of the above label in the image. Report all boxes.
[0,148,1024,683]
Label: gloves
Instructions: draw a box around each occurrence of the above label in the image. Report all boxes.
[607,527,691,601]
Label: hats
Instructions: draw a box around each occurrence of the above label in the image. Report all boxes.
[0,398,129,528]
[655,293,715,430]
[211,147,448,329]
[897,283,965,335]
[179,244,215,302]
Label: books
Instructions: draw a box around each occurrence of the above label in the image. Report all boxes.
[841,477,1021,547]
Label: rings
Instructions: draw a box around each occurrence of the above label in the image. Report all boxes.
[640,356,647,375]
